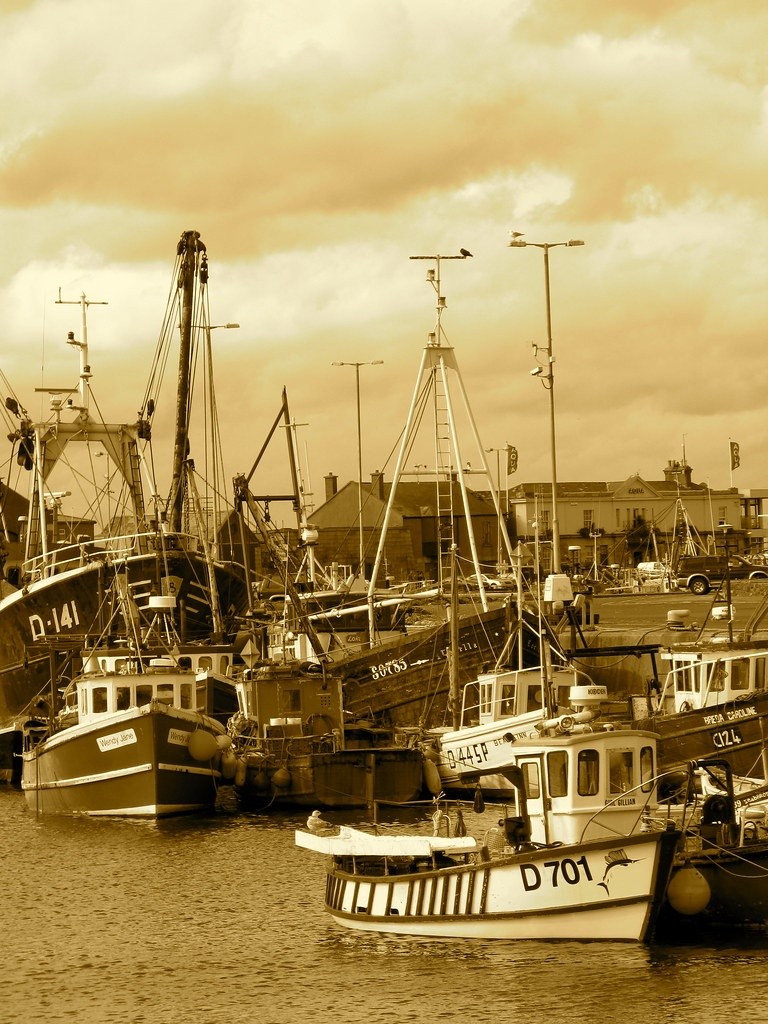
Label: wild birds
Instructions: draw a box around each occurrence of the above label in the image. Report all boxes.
[460,249,472,256]
[306,810,333,830]
[510,231,524,239]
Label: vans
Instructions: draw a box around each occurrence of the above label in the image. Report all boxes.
[637,561,671,573]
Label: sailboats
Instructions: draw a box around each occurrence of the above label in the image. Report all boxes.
[0,229,768,945]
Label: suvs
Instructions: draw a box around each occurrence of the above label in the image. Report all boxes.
[669,553,768,595]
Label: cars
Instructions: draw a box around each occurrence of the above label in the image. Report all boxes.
[437,575,466,590]
[462,572,515,592]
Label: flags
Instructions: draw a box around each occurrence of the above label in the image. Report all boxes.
[508,445,518,475]
[731,442,740,470]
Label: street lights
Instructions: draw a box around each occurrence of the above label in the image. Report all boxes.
[331,359,385,579]
[509,240,583,568]
[176,324,240,559]
